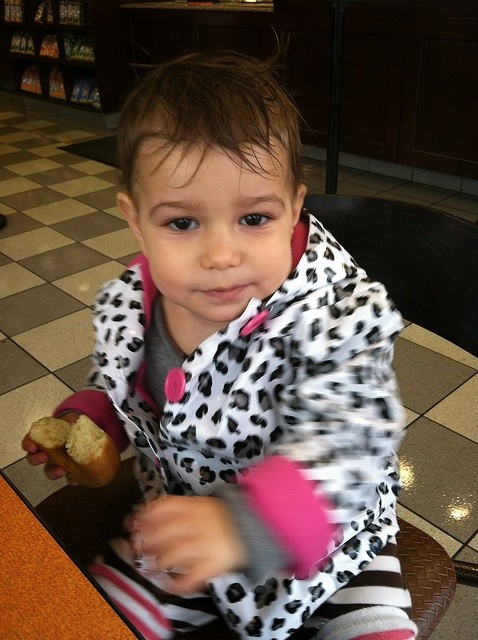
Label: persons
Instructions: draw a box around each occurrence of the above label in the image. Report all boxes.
[20,53,427,635]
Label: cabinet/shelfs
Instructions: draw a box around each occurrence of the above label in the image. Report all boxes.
[398,0,478,180]
[127,8,260,90]
[260,0,398,162]
[1,0,124,114]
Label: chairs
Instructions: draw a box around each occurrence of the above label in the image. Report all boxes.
[35,193,477,640]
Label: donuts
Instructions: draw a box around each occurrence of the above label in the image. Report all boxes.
[27,413,121,489]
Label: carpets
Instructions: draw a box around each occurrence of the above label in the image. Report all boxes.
[56,135,123,171]
